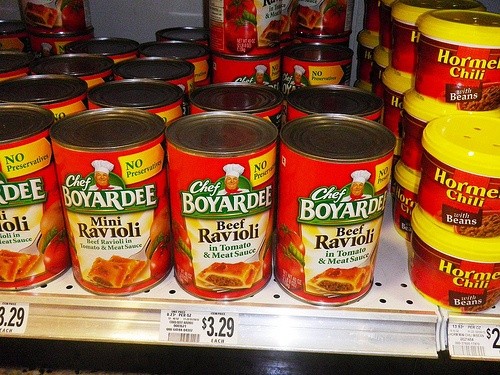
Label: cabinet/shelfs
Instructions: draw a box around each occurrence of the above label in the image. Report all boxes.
[0,192,500,375]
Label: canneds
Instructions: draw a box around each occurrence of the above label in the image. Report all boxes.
[0,0,500,314]
